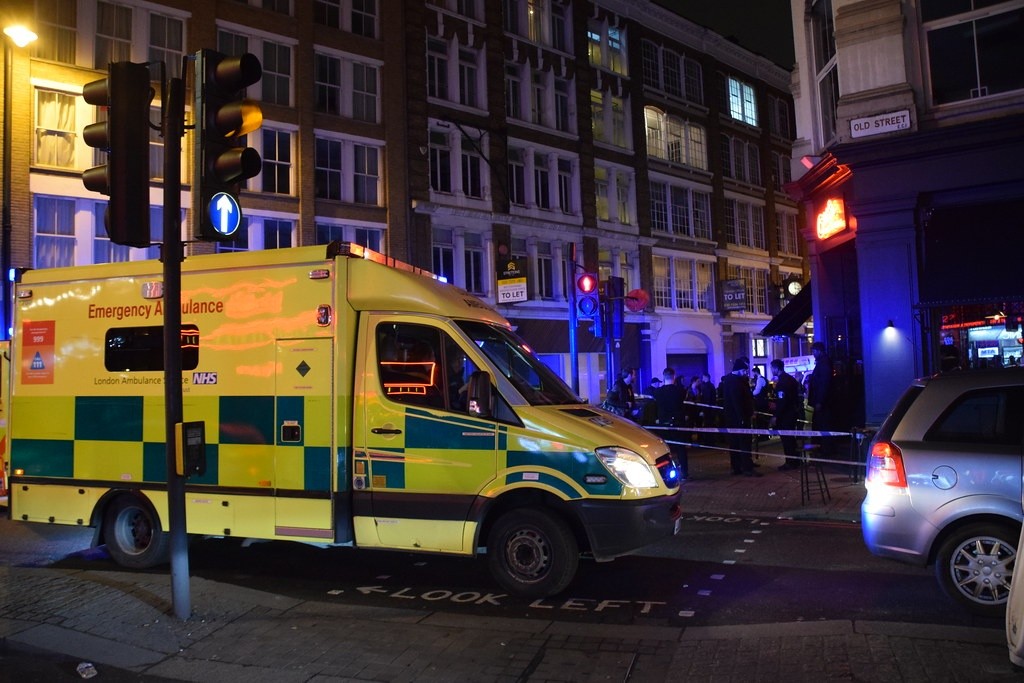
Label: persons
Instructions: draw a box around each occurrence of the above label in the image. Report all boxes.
[980,354,1017,370]
[403,336,470,410]
[807,341,833,458]
[508,356,548,403]
[646,356,807,480]
[601,365,639,424]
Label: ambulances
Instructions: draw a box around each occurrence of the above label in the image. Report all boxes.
[5,239,686,598]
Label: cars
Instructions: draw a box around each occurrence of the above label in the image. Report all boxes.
[859,367,1023,626]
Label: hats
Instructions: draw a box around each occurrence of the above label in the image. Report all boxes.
[732,359,749,372]
[651,378,663,384]
[810,341,826,352]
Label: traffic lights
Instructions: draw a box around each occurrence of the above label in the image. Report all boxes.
[575,273,600,318]
[212,52,267,183]
[78,59,155,247]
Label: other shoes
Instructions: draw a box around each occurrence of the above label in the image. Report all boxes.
[751,462,760,468]
[746,471,764,477]
[778,462,800,471]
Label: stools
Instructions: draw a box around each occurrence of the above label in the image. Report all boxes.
[796,444,832,506]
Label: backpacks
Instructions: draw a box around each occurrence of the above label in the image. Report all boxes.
[848,367,865,405]
[830,367,845,397]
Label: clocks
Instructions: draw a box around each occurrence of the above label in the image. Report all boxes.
[782,272,804,301]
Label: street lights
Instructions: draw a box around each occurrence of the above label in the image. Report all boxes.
[1,22,41,338]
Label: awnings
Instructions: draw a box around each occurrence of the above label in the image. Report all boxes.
[759,276,813,338]
